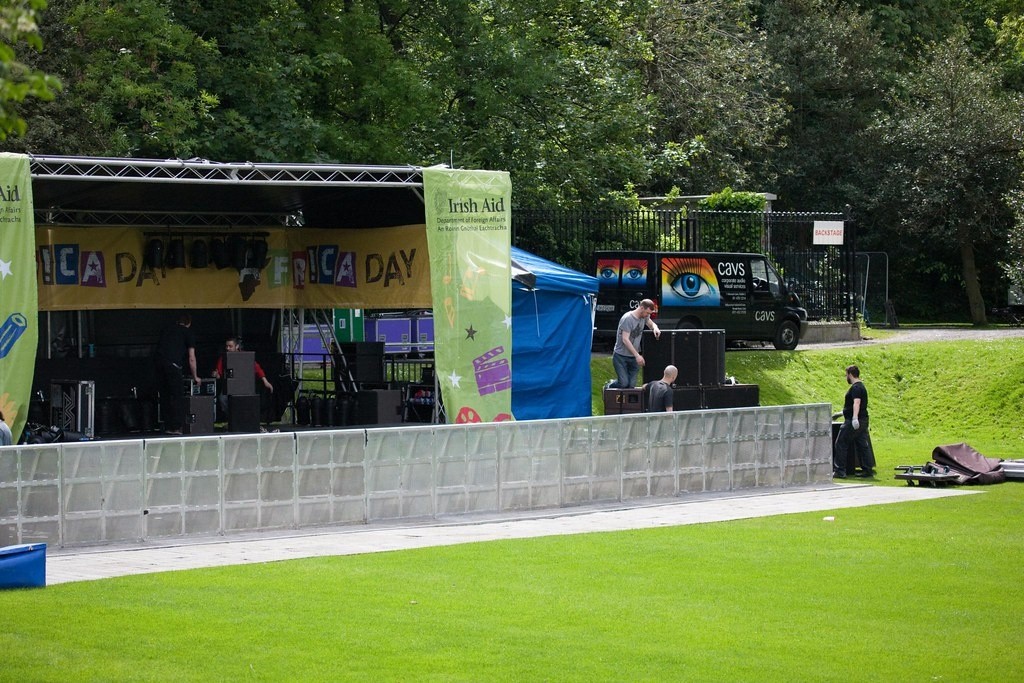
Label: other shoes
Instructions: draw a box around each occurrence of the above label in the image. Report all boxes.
[855,470,872,477]
[166,429,183,435]
[605,379,615,389]
[833,471,846,478]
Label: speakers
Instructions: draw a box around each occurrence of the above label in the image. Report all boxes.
[227,395,261,433]
[181,396,214,436]
[223,350,257,396]
[354,389,402,426]
[602,329,759,416]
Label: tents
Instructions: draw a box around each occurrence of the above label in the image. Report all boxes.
[511,246,598,421]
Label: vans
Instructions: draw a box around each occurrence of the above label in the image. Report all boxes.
[592,249,808,354]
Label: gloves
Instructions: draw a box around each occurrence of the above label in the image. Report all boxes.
[832,412,843,420]
[852,417,859,430]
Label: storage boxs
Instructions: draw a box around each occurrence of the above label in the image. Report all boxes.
[49,378,96,439]
[363,317,412,359]
[602,385,760,415]
[411,315,435,358]
[641,328,726,388]
[282,323,333,363]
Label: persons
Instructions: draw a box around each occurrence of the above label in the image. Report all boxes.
[143,313,202,436]
[602,298,661,401]
[212,337,273,434]
[832,365,876,479]
[642,365,678,413]
[0,411,12,446]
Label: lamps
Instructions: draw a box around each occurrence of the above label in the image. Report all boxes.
[210,235,232,270]
[249,234,269,270]
[166,234,187,269]
[226,233,249,271]
[188,236,208,269]
[142,232,165,270]
